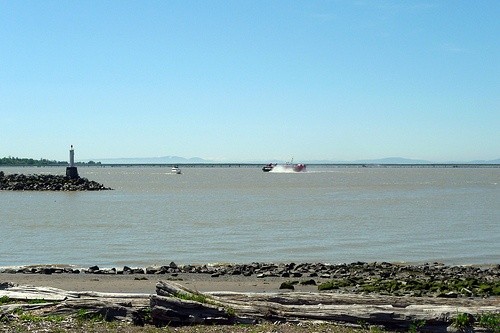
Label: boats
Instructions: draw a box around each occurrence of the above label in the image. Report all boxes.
[262,164,305,172]
[172,168,181,174]
